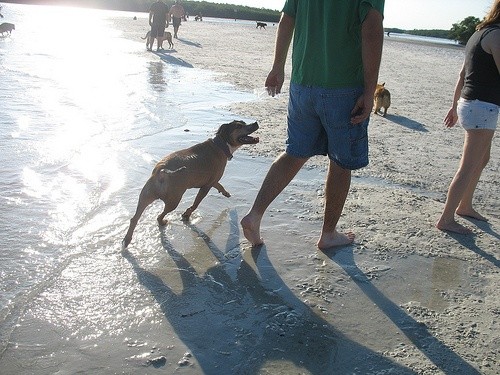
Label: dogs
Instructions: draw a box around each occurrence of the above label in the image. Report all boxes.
[121,120,259,248]
[256,20,267,30]
[373,83,391,117]
[141,31,173,51]
[0,23,16,36]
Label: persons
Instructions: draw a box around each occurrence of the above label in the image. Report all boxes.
[240,0,385,249]
[168,0,184,38]
[437,0,500,235]
[198,10,203,21]
[148,0,170,52]
